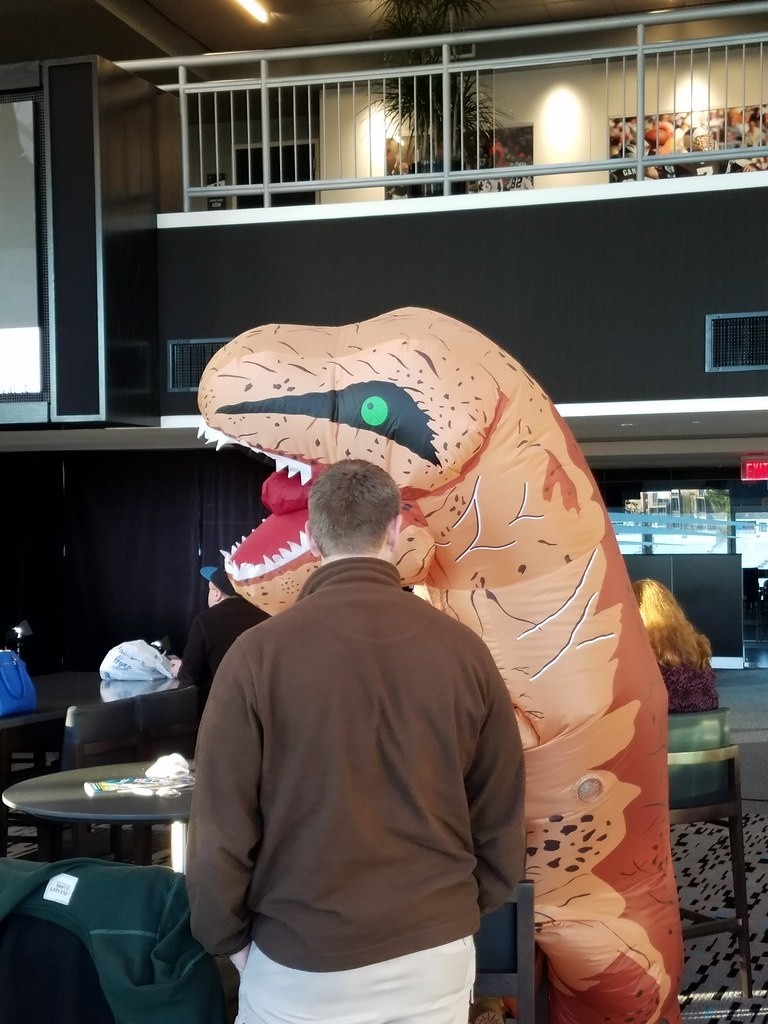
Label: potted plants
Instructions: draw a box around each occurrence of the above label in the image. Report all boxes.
[355,0,513,199]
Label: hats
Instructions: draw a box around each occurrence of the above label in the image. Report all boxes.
[199,566,243,597]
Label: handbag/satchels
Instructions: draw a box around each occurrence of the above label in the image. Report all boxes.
[99,638,176,682]
[0,648,38,719]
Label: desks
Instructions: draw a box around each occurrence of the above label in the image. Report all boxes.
[0,672,193,863]
[2,759,195,862]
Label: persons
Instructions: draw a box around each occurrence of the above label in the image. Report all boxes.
[390,137,534,200]
[609,107,768,183]
[170,566,272,726]
[186,459,527,1024]
[631,578,720,714]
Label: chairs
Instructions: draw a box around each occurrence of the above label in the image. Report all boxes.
[473,880,546,1024]
[0,857,195,1024]
[743,567,766,645]
[1,697,147,863]
[133,685,199,762]
[666,707,752,999]
